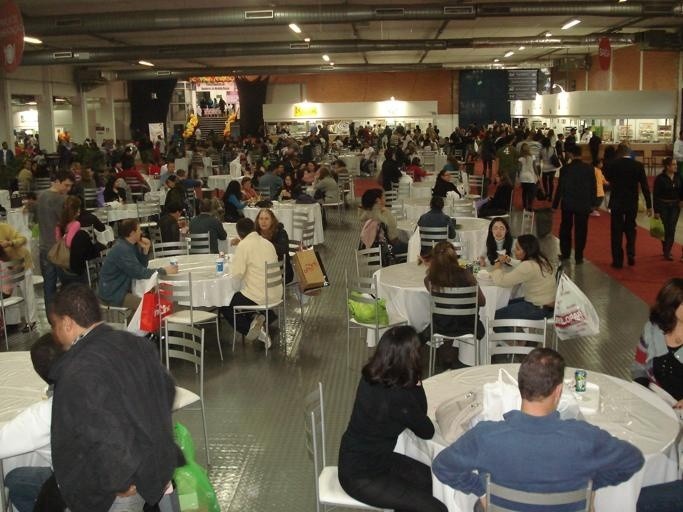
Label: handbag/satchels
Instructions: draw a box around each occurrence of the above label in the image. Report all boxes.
[140,283,173,333]
[649,215,664,240]
[368,222,397,267]
[46,234,70,269]
[435,390,483,444]
[347,289,388,325]
[292,240,330,296]
[537,181,547,200]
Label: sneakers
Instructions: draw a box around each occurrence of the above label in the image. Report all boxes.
[246,314,272,349]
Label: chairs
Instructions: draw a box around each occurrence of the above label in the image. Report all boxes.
[0,266,37,324]
[169,319,212,467]
[85,259,133,327]
[511,264,566,361]
[344,269,408,370]
[2,259,32,348]
[428,281,481,377]
[481,472,594,511]
[354,246,384,290]
[284,236,305,326]
[484,318,548,362]
[232,253,288,356]
[158,273,224,372]
[2,138,682,267]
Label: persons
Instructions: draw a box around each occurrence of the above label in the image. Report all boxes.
[632,275,683,413]
[3,331,67,511]
[1,93,683,368]
[337,323,449,512]
[45,281,188,512]
[431,345,647,512]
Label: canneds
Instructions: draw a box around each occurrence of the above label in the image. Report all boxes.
[472,260,480,273]
[466,264,473,274]
[575,371,586,392]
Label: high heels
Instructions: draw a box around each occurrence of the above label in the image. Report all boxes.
[21,322,36,332]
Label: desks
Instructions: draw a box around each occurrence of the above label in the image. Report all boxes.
[133,253,237,309]
[302,381,381,510]
[391,363,680,512]
[1,351,56,512]
[367,260,523,349]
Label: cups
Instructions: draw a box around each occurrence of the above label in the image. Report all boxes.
[575,369,589,392]
[218,249,225,258]
[170,259,179,274]
[464,258,481,276]
[215,257,225,272]
